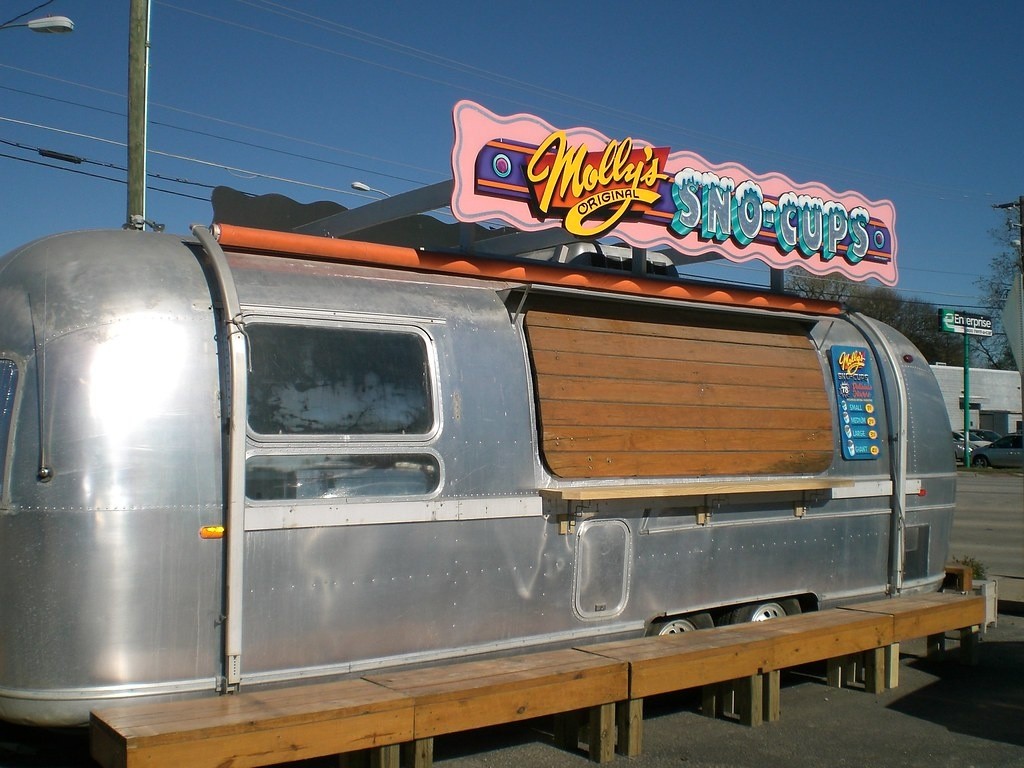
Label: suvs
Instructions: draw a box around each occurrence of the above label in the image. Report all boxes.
[970,432,1024,468]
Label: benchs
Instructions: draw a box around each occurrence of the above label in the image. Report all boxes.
[836,591,987,688]
[89,679,417,768]
[361,648,628,768]
[570,628,773,756]
[716,608,894,722]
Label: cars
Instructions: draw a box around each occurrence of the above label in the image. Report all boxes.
[969,429,1002,442]
[952,431,979,464]
[960,430,993,447]
